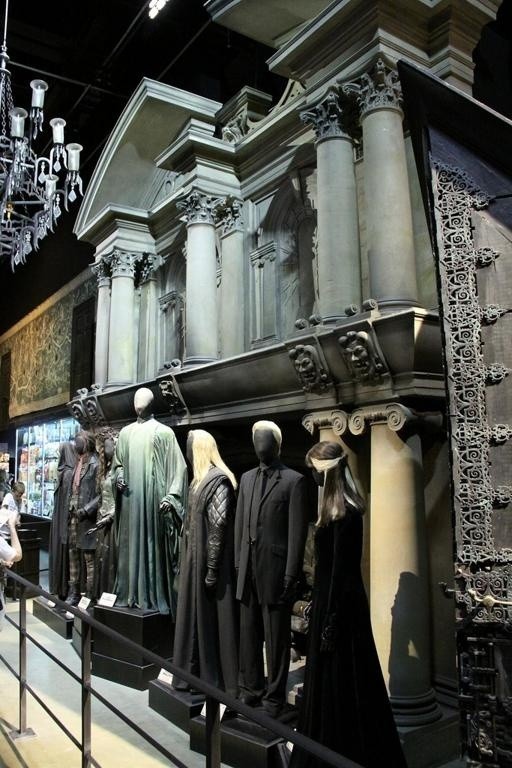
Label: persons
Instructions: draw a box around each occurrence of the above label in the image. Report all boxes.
[0,469,26,514]
[233,420,310,720]
[289,344,332,396]
[337,330,382,386]
[111,387,189,615]
[159,379,184,415]
[0,507,24,633]
[288,441,408,768]
[48,400,120,617]
[170,429,238,697]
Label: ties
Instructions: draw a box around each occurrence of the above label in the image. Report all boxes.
[249,468,265,543]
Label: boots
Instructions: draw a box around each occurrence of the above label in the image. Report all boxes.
[62,580,98,609]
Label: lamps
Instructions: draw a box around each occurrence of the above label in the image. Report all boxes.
[1,1,86,267]
